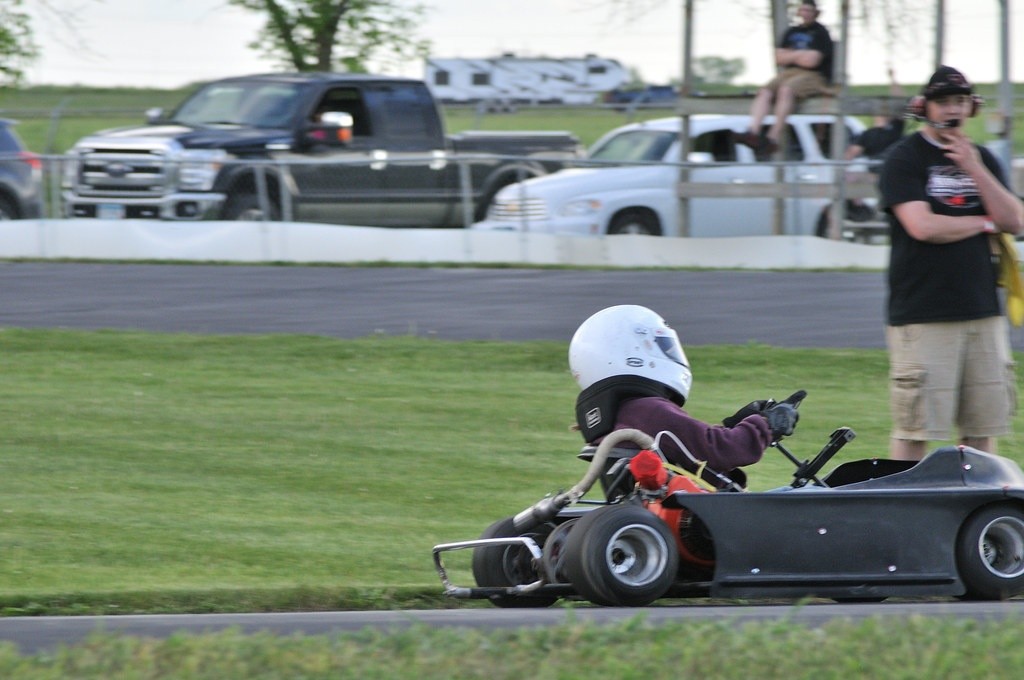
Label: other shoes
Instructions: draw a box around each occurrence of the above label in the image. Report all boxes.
[730,131,777,154]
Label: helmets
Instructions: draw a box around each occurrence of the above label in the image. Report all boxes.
[568,304,691,443]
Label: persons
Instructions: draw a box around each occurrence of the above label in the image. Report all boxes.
[567,304,825,490]
[309,111,352,141]
[733,0,834,150]
[880,68,1024,461]
[843,68,905,222]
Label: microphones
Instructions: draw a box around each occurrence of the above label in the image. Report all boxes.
[925,119,959,128]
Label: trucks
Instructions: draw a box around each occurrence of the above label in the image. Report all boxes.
[424,58,635,104]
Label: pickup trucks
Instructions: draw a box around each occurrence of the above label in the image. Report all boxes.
[607,84,681,113]
[60,72,585,231]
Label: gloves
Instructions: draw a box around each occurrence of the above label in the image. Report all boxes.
[722,399,799,439]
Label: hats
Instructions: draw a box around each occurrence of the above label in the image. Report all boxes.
[924,67,972,96]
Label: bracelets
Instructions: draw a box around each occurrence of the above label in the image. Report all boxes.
[983,215,993,232]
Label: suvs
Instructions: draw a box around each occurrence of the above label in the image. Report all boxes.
[467,114,877,244]
[0,115,47,223]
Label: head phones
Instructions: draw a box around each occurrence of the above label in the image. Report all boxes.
[913,94,982,122]
[797,8,819,16]
